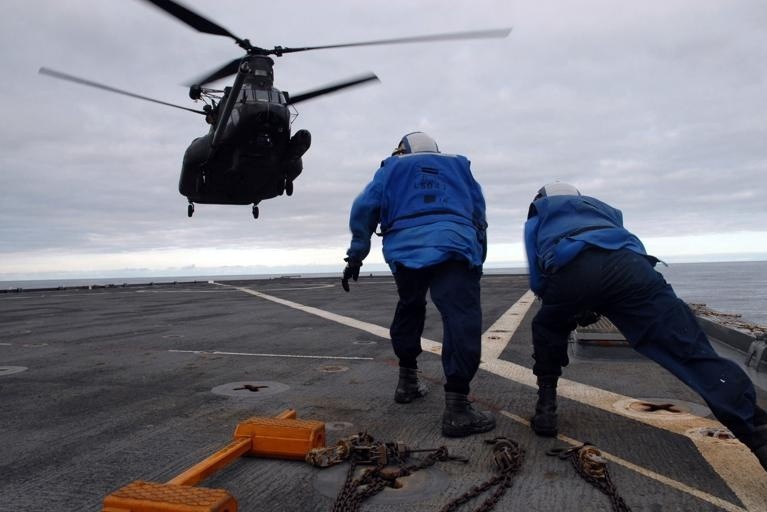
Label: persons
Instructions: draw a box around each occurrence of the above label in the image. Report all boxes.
[521,181,766,472]
[340,130,496,438]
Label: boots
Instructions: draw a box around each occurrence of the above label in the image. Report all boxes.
[442,392,495,438]
[531,388,558,438]
[394,368,432,404]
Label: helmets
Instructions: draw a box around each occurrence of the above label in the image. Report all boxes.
[533,181,581,201]
[392,132,441,156]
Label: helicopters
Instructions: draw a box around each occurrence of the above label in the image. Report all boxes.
[36,0,514,223]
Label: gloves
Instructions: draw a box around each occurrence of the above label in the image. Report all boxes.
[342,257,362,292]
